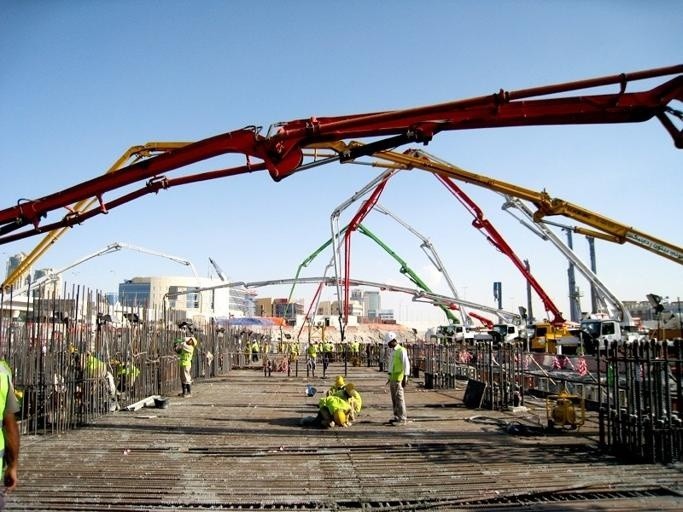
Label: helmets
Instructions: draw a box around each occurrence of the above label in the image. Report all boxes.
[190,336,197,347]
[333,409,346,426]
[334,374,344,386]
[384,331,396,345]
[344,382,356,396]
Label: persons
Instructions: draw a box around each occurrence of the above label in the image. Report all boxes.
[242,338,392,379]
[298,374,363,430]
[173,335,198,398]
[381,331,410,426]
[0,351,20,495]
[63,341,140,413]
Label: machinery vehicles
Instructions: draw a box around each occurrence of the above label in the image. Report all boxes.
[0,64,683,359]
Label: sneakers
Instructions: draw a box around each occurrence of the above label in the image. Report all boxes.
[390,418,406,426]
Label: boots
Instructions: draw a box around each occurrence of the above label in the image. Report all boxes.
[177,383,191,398]
